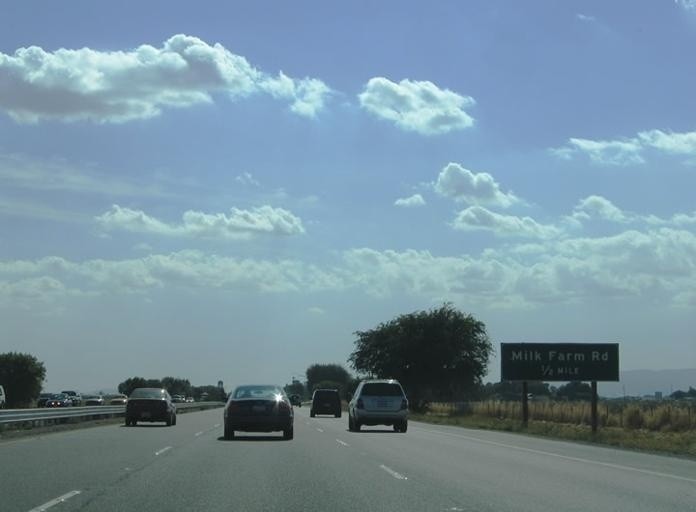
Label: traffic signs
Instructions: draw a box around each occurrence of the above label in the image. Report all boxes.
[500,343,618,381]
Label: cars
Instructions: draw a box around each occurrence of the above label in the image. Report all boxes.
[111,394,128,404]
[37,390,81,408]
[85,394,104,405]
[172,395,195,403]
[291,395,301,407]
[348,379,409,433]
[222,385,294,439]
[125,387,177,425]
[310,389,341,417]
